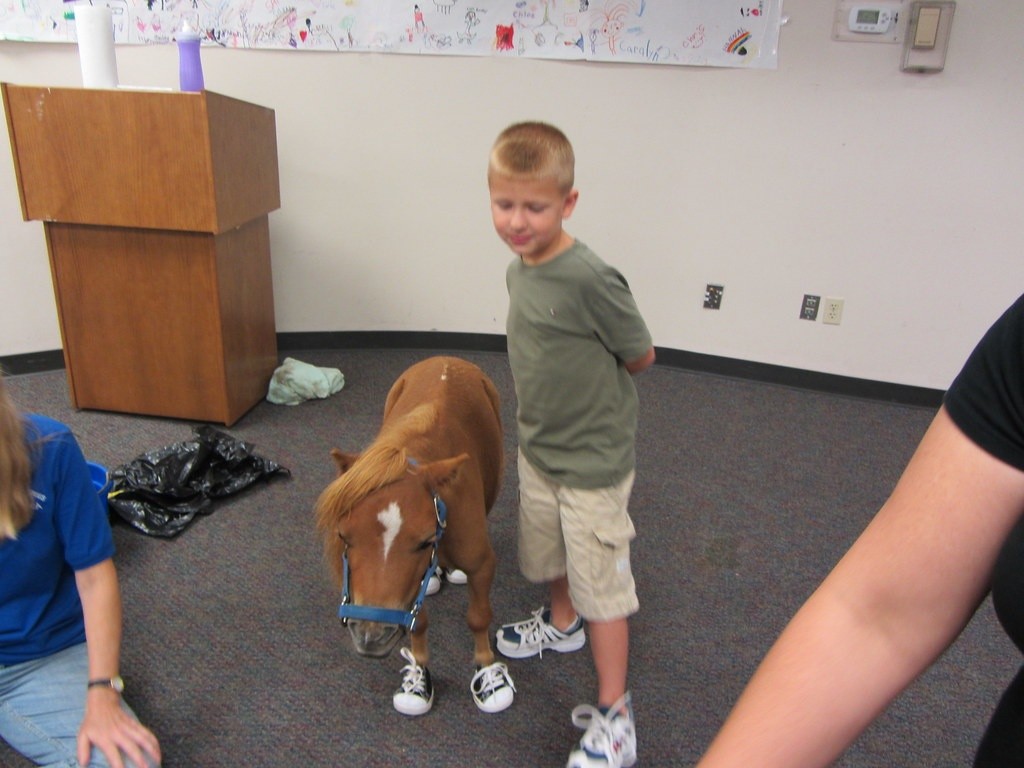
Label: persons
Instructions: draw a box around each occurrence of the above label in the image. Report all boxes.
[0,376,163,768]
[693,289,1023,768]
[491,123,656,767]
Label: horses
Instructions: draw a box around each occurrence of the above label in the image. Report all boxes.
[313,357,516,715]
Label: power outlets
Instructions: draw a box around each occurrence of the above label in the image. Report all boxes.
[823,298,844,326]
[703,284,724,311]
[799,294,821,322]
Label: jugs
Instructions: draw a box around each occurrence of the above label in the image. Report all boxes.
[72,5,118,90]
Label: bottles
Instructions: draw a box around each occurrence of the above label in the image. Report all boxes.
[177,21,204,91]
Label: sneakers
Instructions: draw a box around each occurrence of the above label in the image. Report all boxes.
[420,561,442,596]
[393,647,434,715]
[566,691,637,768]
[444,566,468,584]
[469,662,517,713]
[494,607,586,660]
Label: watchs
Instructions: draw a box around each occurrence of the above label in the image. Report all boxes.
[86,675,124,693]
